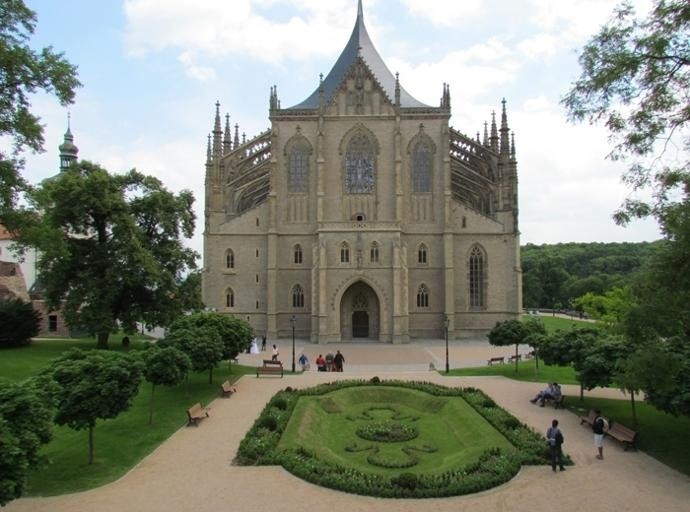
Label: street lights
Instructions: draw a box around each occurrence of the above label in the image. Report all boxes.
[443,314,452,372]
[289,314,298,373]
[203,305,218,384]
[568,295,576,320]
[551,296,556,317]
[527,308,541,370]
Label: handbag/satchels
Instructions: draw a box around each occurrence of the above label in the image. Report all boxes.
[546,438,557,447]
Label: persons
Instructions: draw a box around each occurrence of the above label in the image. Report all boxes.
[245,334,266,353]
[299,353,309,368]
[539,383,561,407]
[547,419,566,471]
[271,344,281,361]
[316,350,345,373]
[530,382,553,403]
[591,410,604,458]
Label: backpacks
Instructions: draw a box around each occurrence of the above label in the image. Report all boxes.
[594,417,611,435]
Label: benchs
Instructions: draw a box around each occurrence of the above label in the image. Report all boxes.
[605,421,637,452]
[221,380,237,398]
[508,354,522,363]
[546,394,565,409]
[525,352,532,359]
[580,409,598,428]
[263,359,283,367]
[256,366,283,378]
[186,403,210,427]
[487,356,504,365]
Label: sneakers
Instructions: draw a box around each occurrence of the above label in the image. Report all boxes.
[552,467,567,472]
[596,454,604,460]
